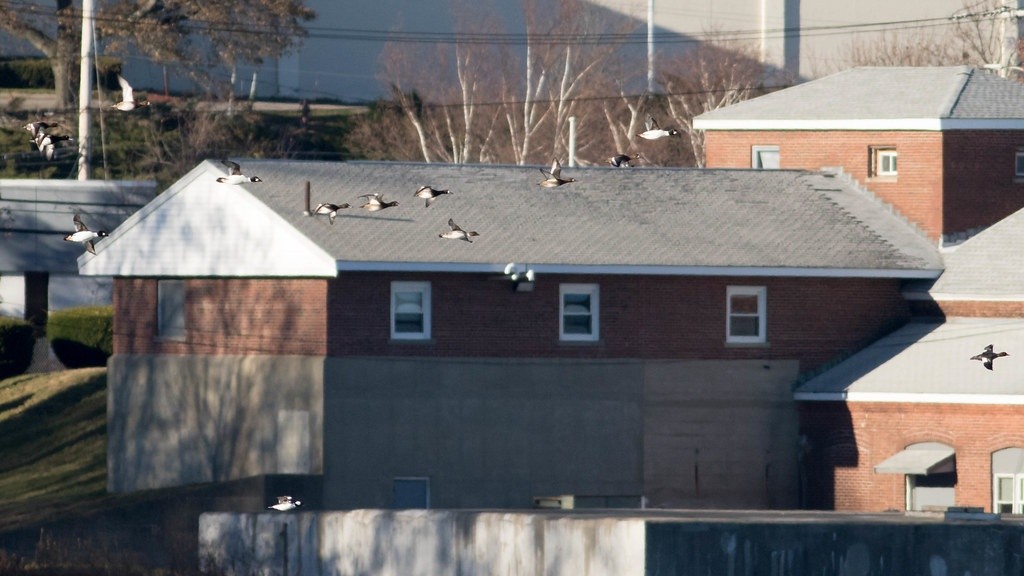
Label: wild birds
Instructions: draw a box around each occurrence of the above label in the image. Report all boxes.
[311,202,353,225]
[538,156,578,189]
[23,121,73,162]
[414,185,454,207]
[110,73,151,113]
[357,193,400,212]
[62,213,109,256]
[439,218,479,243]
[635,117,680,142]
[268,495,305,512]
[216,159,263,184]
[603,152,641,168]
[970,344,1010,371]
